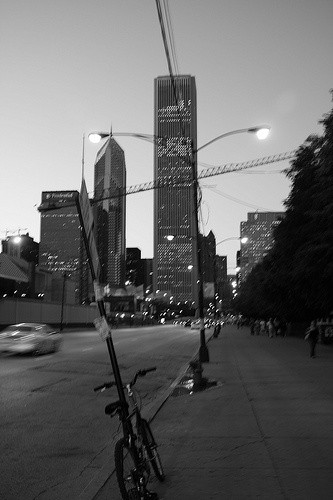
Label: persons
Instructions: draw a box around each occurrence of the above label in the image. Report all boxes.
[249,317,286,339]
[305,321,319,358]
[226,314,249,330]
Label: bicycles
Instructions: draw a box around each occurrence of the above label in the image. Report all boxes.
[93,367,164,500]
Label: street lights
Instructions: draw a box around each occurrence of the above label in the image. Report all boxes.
[90,124,272,363]
[206,237,249,333]
[59,270,72,331]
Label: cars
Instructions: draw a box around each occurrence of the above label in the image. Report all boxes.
[0,322,67,357]
